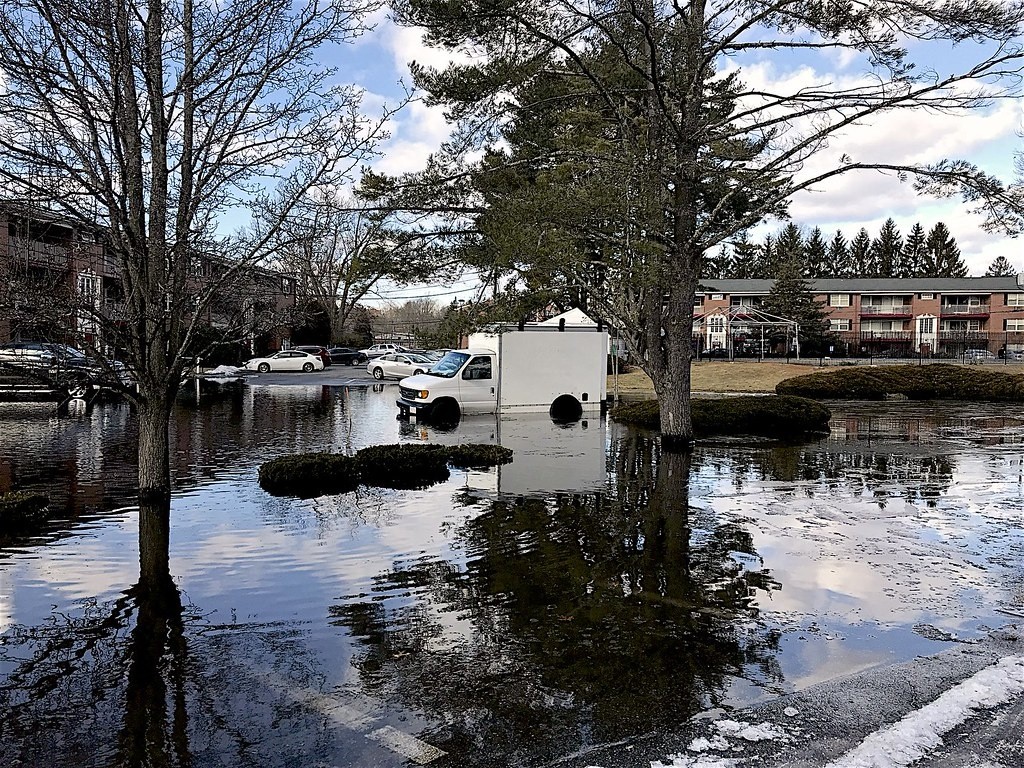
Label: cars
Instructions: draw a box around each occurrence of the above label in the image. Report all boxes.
[1006,350,1023,361]
[0,343,126,398]
[408,346,457,369]
[702,347,738,355]
[398,346,414,353]
[954,349,995,359]
[327,348,368,366]
[245,350,324,373]
[366,353,454,380]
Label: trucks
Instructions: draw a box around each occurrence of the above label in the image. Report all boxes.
[395,320,607,418]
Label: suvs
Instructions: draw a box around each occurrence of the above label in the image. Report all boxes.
[285,346,332,372]
[357,343,401,358]
